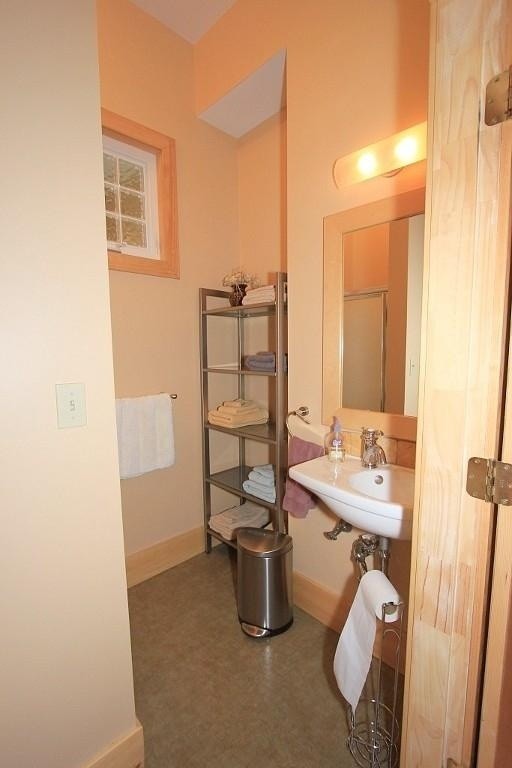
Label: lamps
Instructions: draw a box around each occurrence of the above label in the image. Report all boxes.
[332,122,427,187]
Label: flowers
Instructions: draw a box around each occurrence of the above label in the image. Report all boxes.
[222,267,262,292]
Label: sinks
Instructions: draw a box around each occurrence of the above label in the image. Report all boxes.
[289,454,414,542]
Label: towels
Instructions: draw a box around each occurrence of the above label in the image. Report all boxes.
[207,398,272,429]
[241,285,286,304]
[241,464,286,503]
[115,393,177,481]
[284,435,330,519]
[207,500,288,541]
[244,351,287,374]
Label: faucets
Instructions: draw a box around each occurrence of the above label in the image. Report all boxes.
[360,428,387,468]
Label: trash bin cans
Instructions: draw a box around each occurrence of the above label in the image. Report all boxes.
[236,527,293,638]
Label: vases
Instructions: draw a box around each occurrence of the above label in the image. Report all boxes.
[229,284,247,306]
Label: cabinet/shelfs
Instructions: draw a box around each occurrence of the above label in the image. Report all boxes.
[196,271,288,551]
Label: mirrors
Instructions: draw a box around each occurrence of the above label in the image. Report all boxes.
[321,184,426,442]
